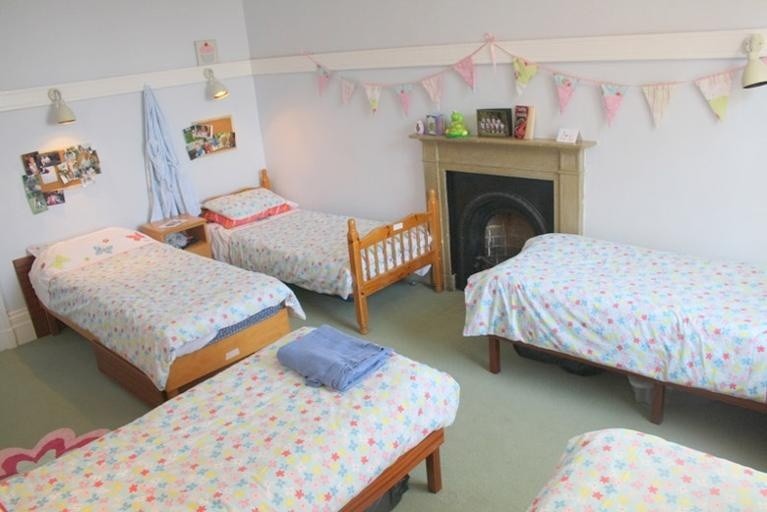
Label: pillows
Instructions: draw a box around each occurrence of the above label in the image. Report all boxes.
[201,186,293,229]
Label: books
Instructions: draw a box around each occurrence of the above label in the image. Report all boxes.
[511,104,538,141]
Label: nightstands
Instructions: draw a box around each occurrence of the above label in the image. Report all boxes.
[139,212,213,260]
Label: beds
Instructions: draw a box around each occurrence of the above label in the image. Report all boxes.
[12,226,308,409]
[1,326,461,511]
[199,168,445,335]
[463,231,766,424]
[528,427,767,512]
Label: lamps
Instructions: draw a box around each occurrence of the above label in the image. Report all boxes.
[203,68,230,102]
[740,36,767,89]
[48,87,77,125]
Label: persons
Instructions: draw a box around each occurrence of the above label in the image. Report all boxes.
[480,116,506,135]
[185,123,235,158]
[23,145,99,211]
